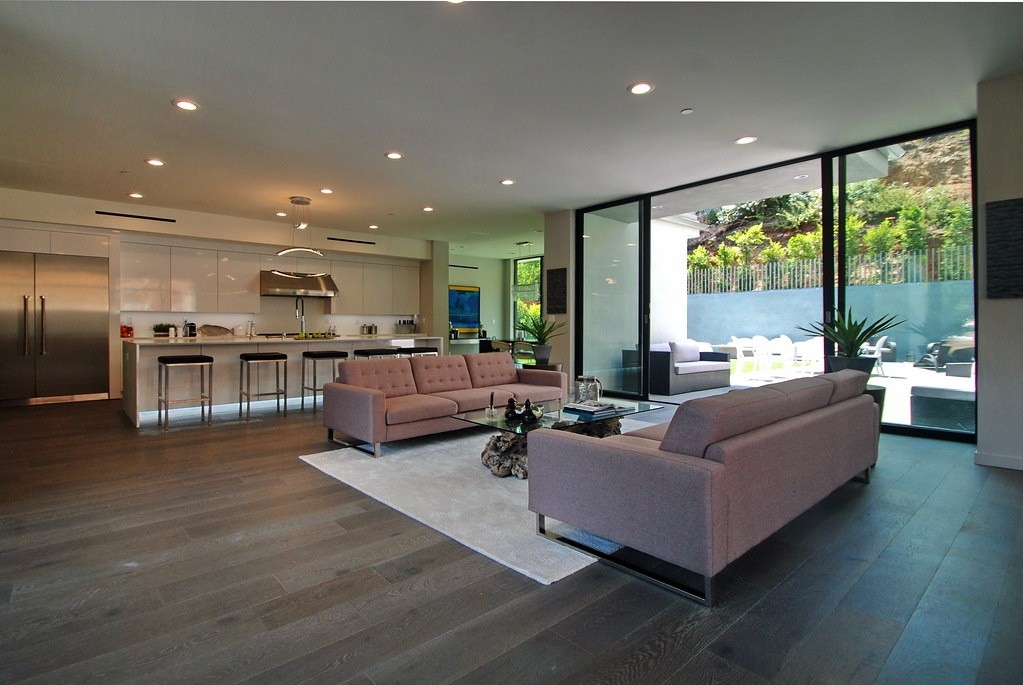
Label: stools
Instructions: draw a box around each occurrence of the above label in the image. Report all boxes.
[354,347,439,358]
[301,351,348,413]
[239,352,287,423]
[157,354,213,431]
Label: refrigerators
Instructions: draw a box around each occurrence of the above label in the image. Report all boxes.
[0,251,110,399]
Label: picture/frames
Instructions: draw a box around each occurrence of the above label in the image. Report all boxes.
[449,285,481,333]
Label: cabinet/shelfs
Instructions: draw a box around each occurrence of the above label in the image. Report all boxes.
[119,241,421,316]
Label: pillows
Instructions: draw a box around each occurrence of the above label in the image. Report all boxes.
[670,338,700,362]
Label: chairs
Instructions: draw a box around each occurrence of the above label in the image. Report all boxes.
[491,340,535,364]
[867,335,896,362]
[913,336,975,371]
[731,333,888,383]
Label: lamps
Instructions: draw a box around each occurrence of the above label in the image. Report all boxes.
[271,196,326,278]
[512,242,540,301]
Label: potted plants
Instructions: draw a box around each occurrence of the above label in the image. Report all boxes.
[513,314,568,364]
[795,305,907,372]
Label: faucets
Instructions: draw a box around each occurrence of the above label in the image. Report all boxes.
[295,297,305,336]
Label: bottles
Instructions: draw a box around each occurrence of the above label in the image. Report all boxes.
[169,328,174,337]
[370,324,377,334]
[484,406,496,420]
[575,375,599,401]
[361,323,368,334]
[177,328,183,337]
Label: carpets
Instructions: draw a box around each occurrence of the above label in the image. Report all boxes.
[298,417,660,586]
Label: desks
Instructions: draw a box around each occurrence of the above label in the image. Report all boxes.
[479,339,538,353]
[448,338,490,355]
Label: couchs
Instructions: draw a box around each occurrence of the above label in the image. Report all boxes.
[622,343,730,395]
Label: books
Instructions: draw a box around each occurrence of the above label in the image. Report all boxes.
[563,409,615,417]
[564,399,635,414]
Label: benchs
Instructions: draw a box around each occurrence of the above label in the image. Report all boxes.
[527,368,880,608]
[910,362,975,432]
[322,352,568,460]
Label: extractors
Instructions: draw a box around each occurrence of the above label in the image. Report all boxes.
[260,270,340,298]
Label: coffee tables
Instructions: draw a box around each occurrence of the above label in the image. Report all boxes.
[449,397,664,479]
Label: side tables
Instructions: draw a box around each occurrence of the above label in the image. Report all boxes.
[865,384,886,422]
[522,364,563,373]
